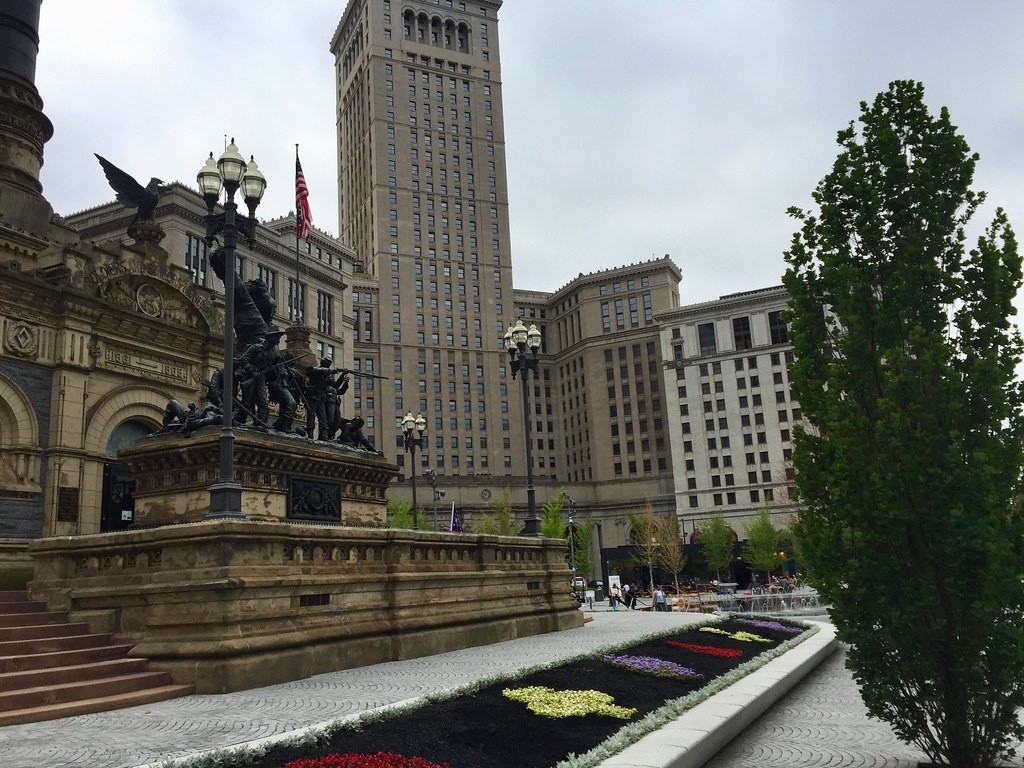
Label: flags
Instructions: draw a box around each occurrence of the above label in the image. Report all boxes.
[452,506,463,532]
[296,150,312,239]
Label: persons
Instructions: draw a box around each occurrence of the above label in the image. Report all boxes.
[780,575,787,589]
[624,582,638,611]
[652,584,667,612]
[609,583,619,608]
[158,329,377,451]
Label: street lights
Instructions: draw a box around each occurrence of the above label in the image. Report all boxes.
[195,133,272,520]
[396,409,428,532]
[501,317,542,536]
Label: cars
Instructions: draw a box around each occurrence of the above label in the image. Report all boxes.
[570,577,587,591]
